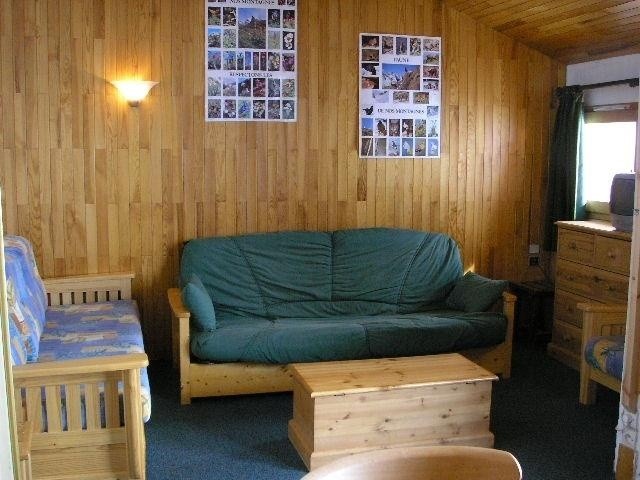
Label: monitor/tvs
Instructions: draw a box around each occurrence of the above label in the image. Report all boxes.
[608,174,635,233]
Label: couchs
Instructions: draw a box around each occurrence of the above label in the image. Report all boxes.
[165,228,518,404]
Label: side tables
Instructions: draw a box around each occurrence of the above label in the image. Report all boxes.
[508,280,555,349]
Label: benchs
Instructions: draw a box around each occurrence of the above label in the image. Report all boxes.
[1,233,152,463]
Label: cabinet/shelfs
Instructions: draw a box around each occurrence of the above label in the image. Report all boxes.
[547,217,634,376]
